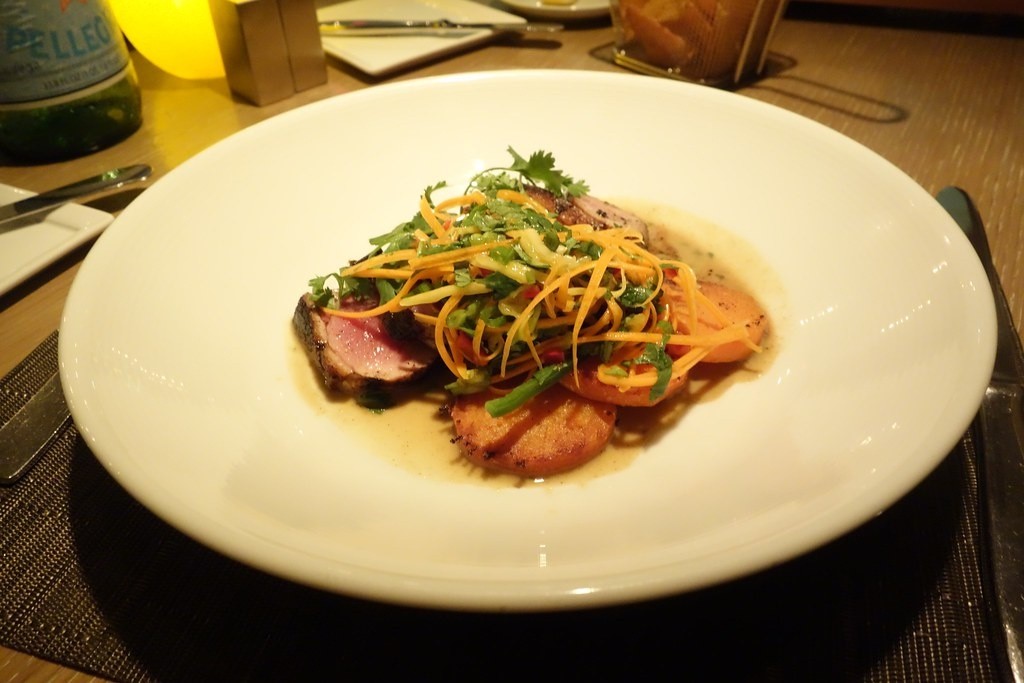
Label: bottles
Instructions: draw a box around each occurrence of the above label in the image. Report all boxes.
[0,1,144,167]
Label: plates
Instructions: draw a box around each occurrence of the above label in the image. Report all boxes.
[0,179,114,298]
[58,68,999,613]
[313,1,528,79]
[501,0,613,20]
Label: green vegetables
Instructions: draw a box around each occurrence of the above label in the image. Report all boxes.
[305,146,674,417]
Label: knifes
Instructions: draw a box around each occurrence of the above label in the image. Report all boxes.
[930,184,1024,683]
[314,15,567,38]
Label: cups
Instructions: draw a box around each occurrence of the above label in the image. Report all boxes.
[608,1,786,87]
